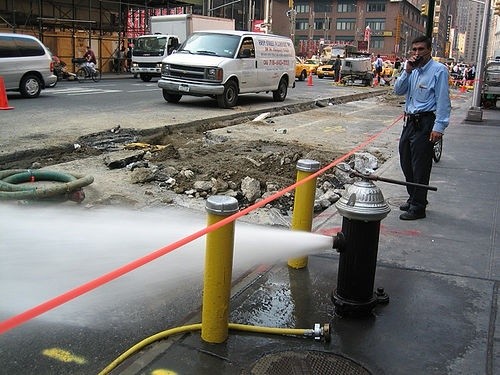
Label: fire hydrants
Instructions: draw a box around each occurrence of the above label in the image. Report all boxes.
[329,162,392,320]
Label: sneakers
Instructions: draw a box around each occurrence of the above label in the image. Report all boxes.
[399,202,410,211]
[400,210,426,220]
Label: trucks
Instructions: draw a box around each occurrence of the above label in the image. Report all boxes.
[480,56,500,108]
[131,13,237,83]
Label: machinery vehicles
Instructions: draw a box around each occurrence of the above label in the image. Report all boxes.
[339,52,374,86]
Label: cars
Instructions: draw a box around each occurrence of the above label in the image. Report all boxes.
[295,56,311,82]
[301,58,321,73]
[317,57,345,79]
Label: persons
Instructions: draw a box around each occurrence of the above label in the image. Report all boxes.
[394,36,451,220]
[332,55,341,82]
[117,48,126,72]
[374,54,383,83]
[127,46,132,72]
[452,64,476,80]
[83,47,97,76]
[392,55,410,78]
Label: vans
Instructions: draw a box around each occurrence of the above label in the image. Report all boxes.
[0,32,57,98]
[453,63,468,72]
[157,28,298,105]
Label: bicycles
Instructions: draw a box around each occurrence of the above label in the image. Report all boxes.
[76,58,103,83]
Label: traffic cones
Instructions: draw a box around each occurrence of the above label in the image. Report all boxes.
[306,71,315,86]
[0,75,15,111]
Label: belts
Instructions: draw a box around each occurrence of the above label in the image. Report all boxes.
[406,111,433,122]
